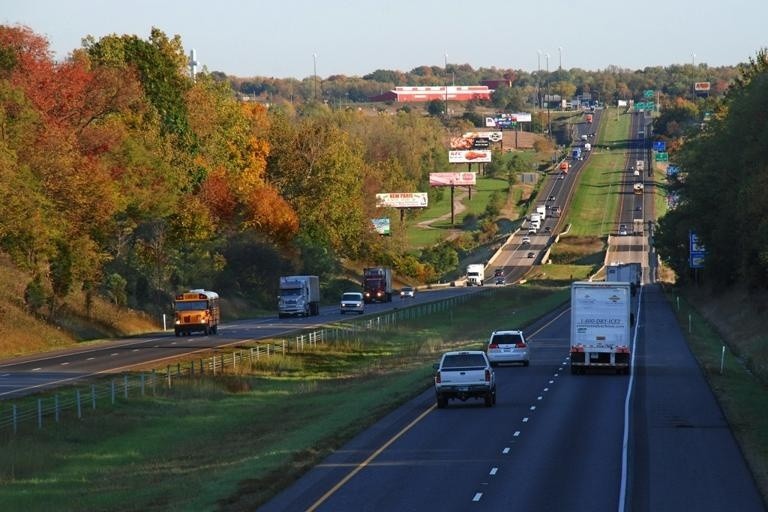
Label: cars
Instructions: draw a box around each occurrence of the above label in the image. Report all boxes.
[548,195,556,200]
[557,175,564,180]
[493,268,506,285]
[629,165,639,177]
[620,224,633,236]
[522,227,551,259]
[400,287,414,298]
[340,292,365,314]
[433,329,530,409]
[545,204,551,209]
[636,205,641,210]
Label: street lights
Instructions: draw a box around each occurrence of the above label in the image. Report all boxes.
[690,52,695,104]
[312,51,318,100]
[536,46,563,137]
[444,54,448,115]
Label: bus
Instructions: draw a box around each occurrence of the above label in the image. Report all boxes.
[170,289,220,336]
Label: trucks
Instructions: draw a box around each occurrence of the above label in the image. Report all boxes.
[560,162,568,174]
[633,183,644,194]
[530,204,545,230]
[633,219,644,236]
[585,143,591,151]
[635,161,644,171]
[586,115,592,123]
[277,275,320,318]
[466,263,484,287]
[573,148,580,160]
[361,266,393,304]
[569,261,642,376]
